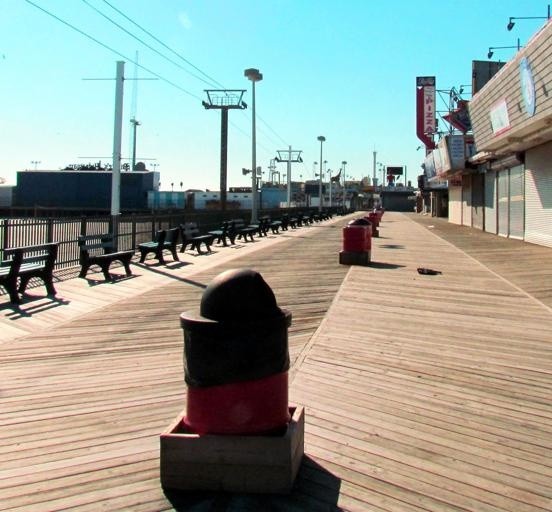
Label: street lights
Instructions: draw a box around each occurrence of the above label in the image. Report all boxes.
[341,161,348,206]
[244,67,263,226]
[316,135,327,211]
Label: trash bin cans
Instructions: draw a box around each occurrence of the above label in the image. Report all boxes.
[339,207,385,266]
[160,268,305,494]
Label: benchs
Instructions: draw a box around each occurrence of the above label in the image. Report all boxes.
[209,206,355,246]
[137,228,181,265]
[1,240,61,306]
[74,233,136,281]
[179,221,213,255]
